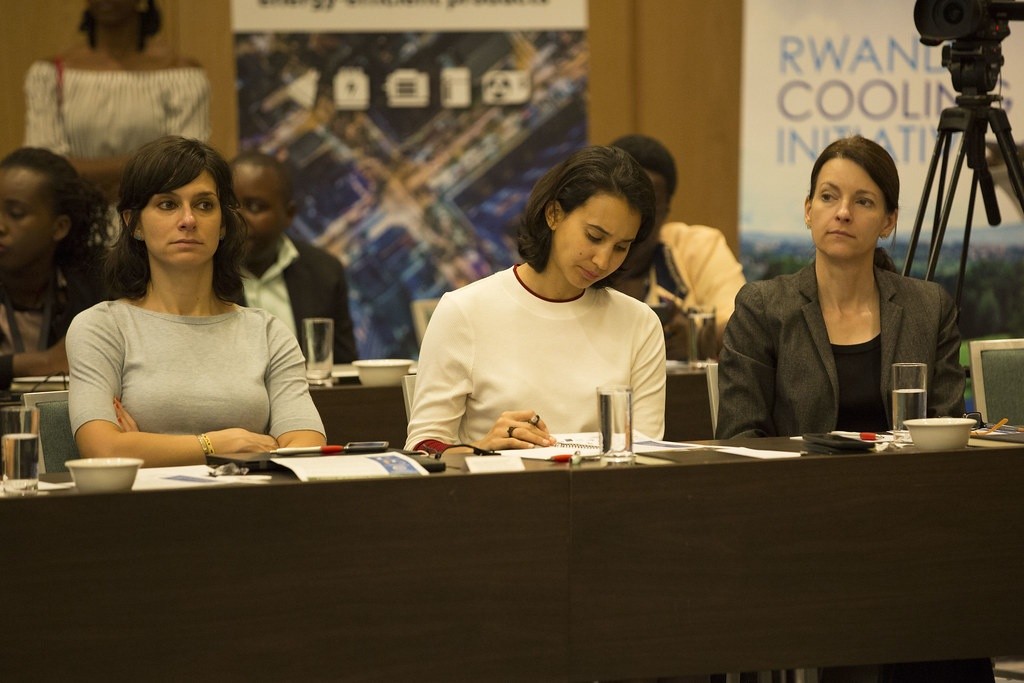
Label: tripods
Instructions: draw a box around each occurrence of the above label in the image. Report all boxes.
[901,41,1024,325]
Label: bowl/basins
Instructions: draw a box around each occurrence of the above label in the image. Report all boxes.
[352,358,413,388]
[903,418,977,451]
[65,458,143,493]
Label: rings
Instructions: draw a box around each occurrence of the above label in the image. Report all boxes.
[507,427,516,437]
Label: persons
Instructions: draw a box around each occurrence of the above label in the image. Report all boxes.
[0,144,109,377]
[25,0,212,204]
[64,134,328,469]
[403,145,667,452]
[714,135,967,440]
[605,133,748,363]
[227,151,361,383]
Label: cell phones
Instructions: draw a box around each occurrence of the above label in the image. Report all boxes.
[346,442,388,453]
[803,433,874,450]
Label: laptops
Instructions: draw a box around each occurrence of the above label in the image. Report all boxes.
[207,448,424,472]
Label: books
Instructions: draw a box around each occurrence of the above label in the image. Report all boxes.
[490,431,626,460]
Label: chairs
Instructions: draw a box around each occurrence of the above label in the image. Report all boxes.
[21,390,78,478]
[968,339,1024,434]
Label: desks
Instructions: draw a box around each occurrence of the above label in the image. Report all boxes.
[0,376,712,455]
[0,426,1024,683]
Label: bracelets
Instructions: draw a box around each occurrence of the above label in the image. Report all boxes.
[196,432,214,456]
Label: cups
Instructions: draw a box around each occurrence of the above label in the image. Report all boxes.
[687,305,715,370]
[596,383,636,463]
[302,318,333,389]
[891,363,927,442]
[0,406,42,498]
[0,349,14,400]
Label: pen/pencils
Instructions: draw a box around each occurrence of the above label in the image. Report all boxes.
[269,445,346,456]
[828,430,885,441]
[528,414,540,426]
